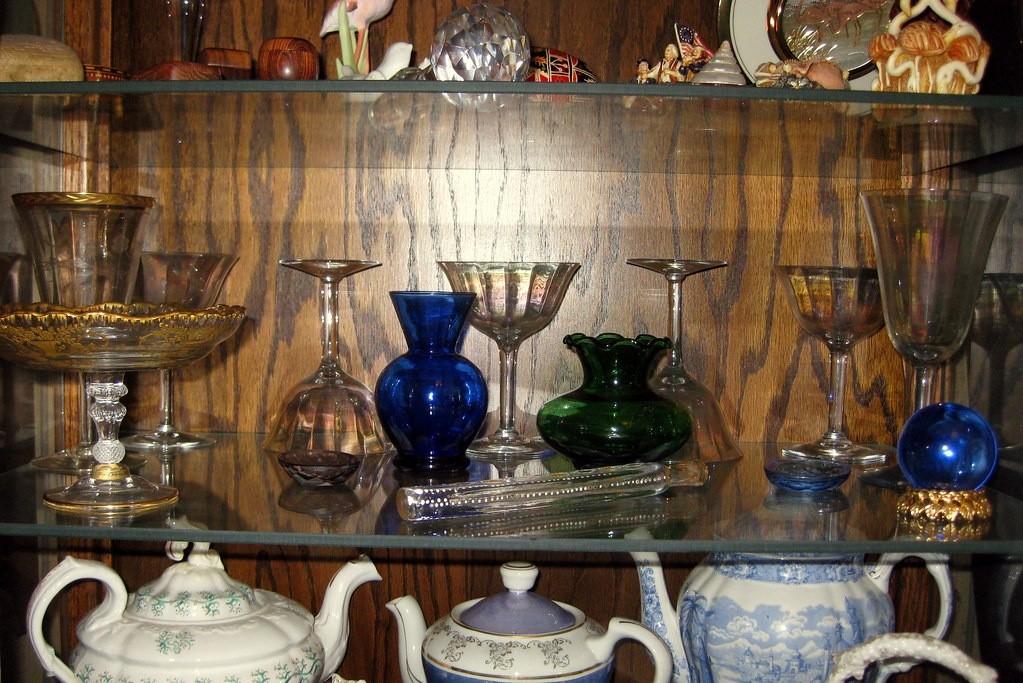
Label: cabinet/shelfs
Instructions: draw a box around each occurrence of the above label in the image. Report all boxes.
[1,1,1022,683]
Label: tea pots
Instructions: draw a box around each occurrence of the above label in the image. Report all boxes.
[385,561,673,683]
[25,517,382,683]
[624,497,954,683]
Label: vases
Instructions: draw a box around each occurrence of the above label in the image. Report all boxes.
[375,291,490,474]
[535,332,693,470]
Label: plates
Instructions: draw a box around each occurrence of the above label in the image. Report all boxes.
[278,451,360,485]
[764,460,851,491]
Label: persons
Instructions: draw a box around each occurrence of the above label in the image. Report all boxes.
[642,44,685,83]
[631,58,657,85]
[678,46,708,83]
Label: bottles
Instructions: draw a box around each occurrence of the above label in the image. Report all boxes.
[536,333,694,470]
[375,291,488,472]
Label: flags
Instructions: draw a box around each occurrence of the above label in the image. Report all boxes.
[674,21,715,65]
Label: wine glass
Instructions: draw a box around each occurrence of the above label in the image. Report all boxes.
[626,258,744,464]
[116,249,241,447]
[259,259,396,454]
[0,298,247,516]
[969,271,1023,451]
[12,192,159,471]
[436,261,581,459]
[856,188,1010,489]
[771,265,896,465]
